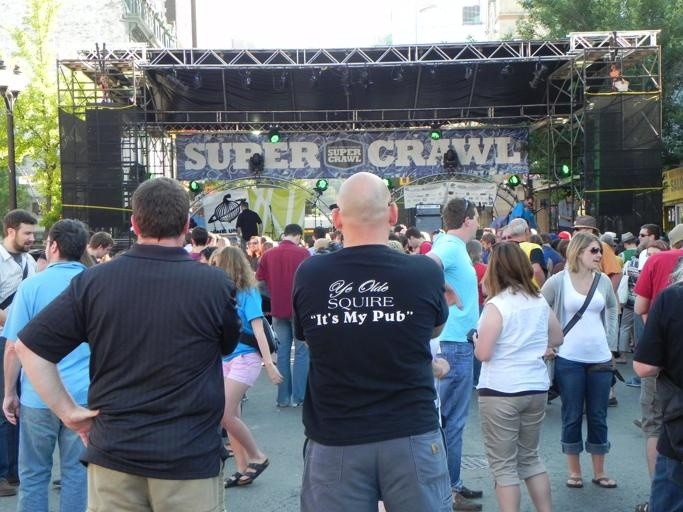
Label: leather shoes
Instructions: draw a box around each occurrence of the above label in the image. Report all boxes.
[458,487,482,499]
[453,493,482,512]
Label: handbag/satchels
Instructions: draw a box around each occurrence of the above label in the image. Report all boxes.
[256,316,279,357]
[617,275,629,305]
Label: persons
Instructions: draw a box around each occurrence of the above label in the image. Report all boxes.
[14,177,242,512]
[0,218,89,511]
[631,281,683,512]
[423,195,485,512]
[0,207,39,496]
[631,222,683,512]
[207,245,285,488]
[31,188,683,409]
[473,239,563,512]
[539,230,620,489]
[291,170,453,512]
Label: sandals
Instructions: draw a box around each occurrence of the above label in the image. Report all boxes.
[636,503,650,512]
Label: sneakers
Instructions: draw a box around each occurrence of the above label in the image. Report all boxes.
[1,480,16,497]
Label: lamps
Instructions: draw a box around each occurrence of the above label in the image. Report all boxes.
[431,130,441,140]
[561,162,570,177]
[508,175,521,186]
[382,178,392,190]
[189,180,202,192]
[268,130,281,144]
[313,180,328,197]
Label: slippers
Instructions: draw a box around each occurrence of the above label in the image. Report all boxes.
[567,476,583,488]
[224,458,269,489]
[592,477,617,489]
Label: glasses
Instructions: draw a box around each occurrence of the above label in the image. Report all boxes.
[584,247,603,255]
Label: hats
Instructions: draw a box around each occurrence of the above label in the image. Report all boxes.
[622,232,634,242]
[574,216,600,232]
